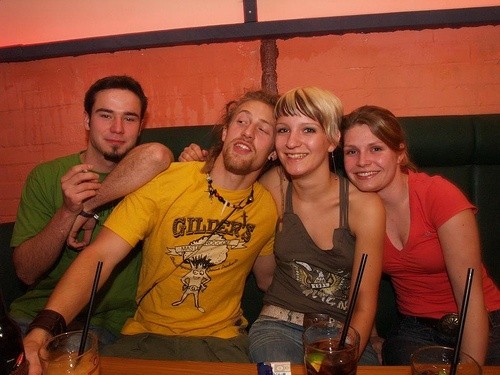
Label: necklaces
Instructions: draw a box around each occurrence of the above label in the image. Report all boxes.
[204,169,253,216]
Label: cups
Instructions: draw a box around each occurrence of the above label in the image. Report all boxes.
[303,320,361,375]
[411,345,483,375]
[37,330,101,375]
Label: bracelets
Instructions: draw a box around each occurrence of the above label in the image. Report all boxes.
[27,309,67,338]
[80,210,99,220]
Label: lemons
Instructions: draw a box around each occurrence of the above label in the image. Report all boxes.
[306,353,325,373]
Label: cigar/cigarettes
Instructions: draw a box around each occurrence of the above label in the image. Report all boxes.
[82,167,87,172]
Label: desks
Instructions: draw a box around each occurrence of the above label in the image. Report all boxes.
[11,348,500,375]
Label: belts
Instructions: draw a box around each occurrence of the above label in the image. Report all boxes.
[260,305,345,330]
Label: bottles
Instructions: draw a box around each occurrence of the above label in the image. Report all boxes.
[0,284,28,375]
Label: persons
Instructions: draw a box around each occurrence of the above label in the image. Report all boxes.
[0,74,175,375]
[23,87,282,375]
[339,104,500,375]
[178,87,385,363]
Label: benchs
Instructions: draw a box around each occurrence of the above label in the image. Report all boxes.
[131,113,500,346]
